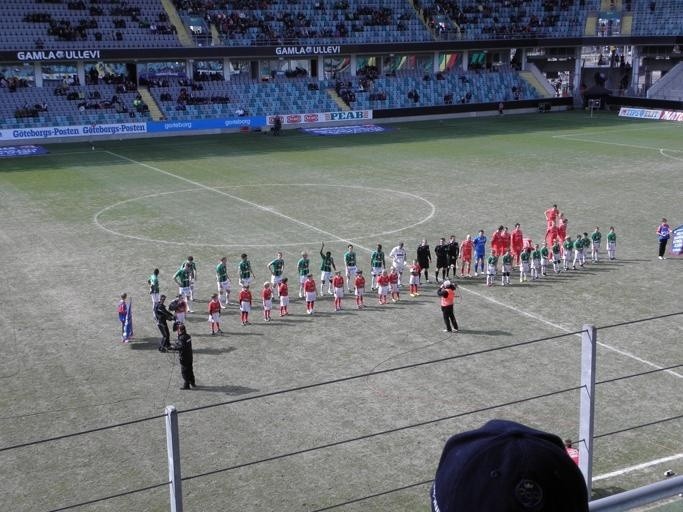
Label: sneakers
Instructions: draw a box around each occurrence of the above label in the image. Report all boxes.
[441,326,459,334]
[335,305,369,311]
[209,328,224,338]
[410,292,420,298]
[378,297,401,305]
[219,301,235,308]
[485,281,514,288]
[657,254,667,261]
[158,343,177,354]
[240,320,252,326]
[460,272,485,279]
[263,312,291,323]
[176,381,198,392]
[517,268,565,284]
[569,257,618,269]
[320,290,334,298]
[305,308,317,315]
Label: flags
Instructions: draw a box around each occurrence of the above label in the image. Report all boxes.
[121,302,134,342]
[668,225,683,256]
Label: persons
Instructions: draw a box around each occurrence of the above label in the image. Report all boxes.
[204,0,454,106]
[170,324,195,390]
[607,226,616,260]
[565,439,578,466]
[118,292,127,337]
[1,1,231,120]
[656,217,673,260]
[591,226,601,263]
[147,242,408,353]
[410,204,591,297]
[413,1,657,117]
[437,281,459,332]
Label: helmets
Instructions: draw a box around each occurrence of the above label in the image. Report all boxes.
[443,280,452,289]
